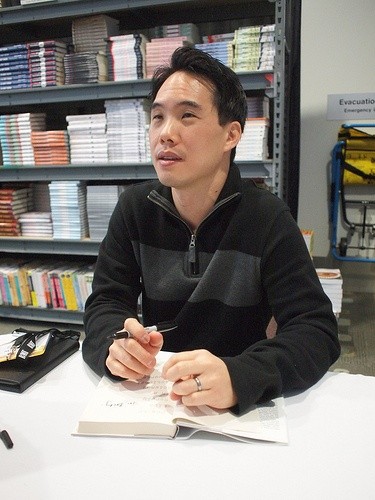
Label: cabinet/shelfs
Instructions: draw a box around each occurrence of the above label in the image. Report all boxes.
[0,0,300,339]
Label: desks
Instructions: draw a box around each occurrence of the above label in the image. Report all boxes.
[0,334,375,500]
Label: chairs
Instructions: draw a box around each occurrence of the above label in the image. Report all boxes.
[329,122,375,263]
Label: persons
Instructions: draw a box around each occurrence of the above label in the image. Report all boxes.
[81,46,342,416]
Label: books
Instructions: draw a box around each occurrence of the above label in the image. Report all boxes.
[72,351,289,448]
[0,255,98,314]
[0,16,278,89]
[300,228,344,319]
[0,181,130,242]
[0,97,271,166]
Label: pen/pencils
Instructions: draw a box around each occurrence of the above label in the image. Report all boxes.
[107,321,178,340]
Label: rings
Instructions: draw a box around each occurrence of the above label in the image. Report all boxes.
[194,376,202,392]
